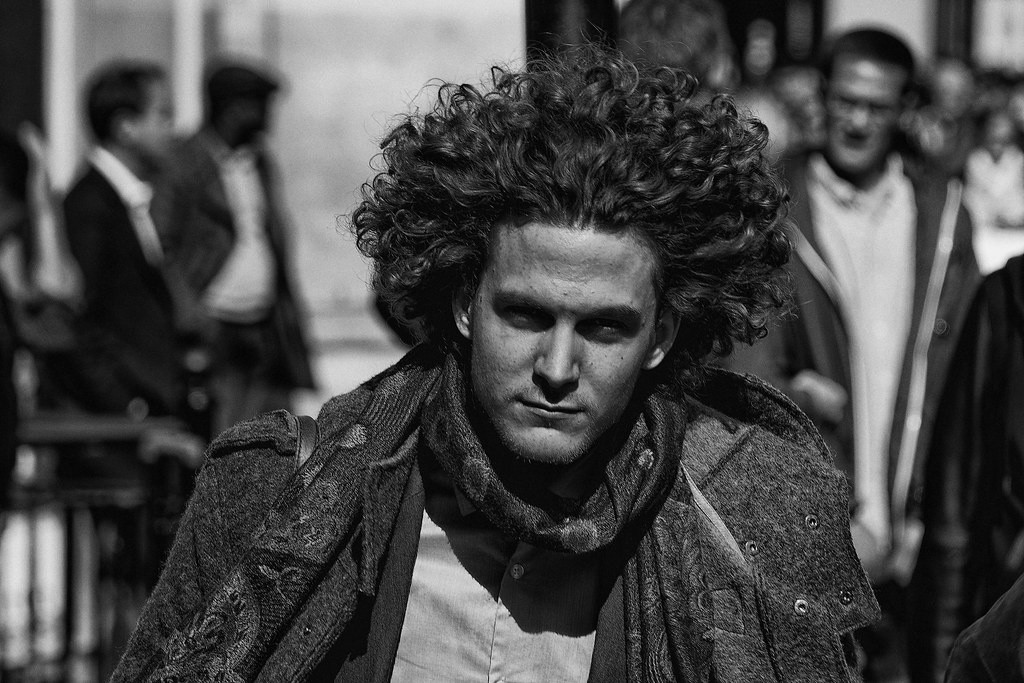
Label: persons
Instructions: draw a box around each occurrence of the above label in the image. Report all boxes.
[110,47,884,683]
[0,58,320,683]
[618,0,1024,683]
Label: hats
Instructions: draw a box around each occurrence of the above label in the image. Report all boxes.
[208,67,278,102]
